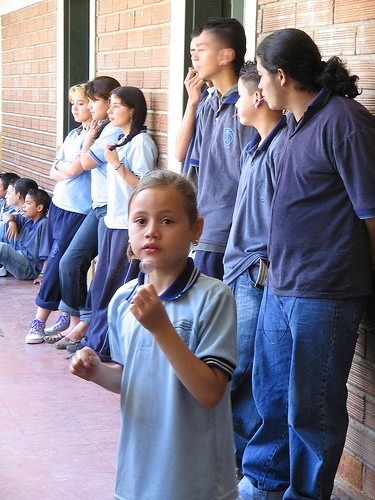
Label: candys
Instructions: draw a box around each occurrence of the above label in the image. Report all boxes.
[139,259,154,284]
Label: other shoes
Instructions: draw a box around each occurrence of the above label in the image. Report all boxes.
[0,265,7,276]
[66,343,84,352]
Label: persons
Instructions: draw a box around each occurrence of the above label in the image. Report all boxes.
[174,18,258,284]
[221,60,288,479]
[0,172,50,286]
[68,169,239,500]
[26,75,160,362]
[234,27,375,500]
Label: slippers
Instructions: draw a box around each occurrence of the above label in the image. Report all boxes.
[44,333,64,343]
[54,336,80,350]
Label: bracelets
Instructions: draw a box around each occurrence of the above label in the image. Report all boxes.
[54,159,63,170]
[114,162,123,170]
[39,272,44,277]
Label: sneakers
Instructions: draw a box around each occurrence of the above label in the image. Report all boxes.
[44,313,70,334]
[25,319,46,344]
